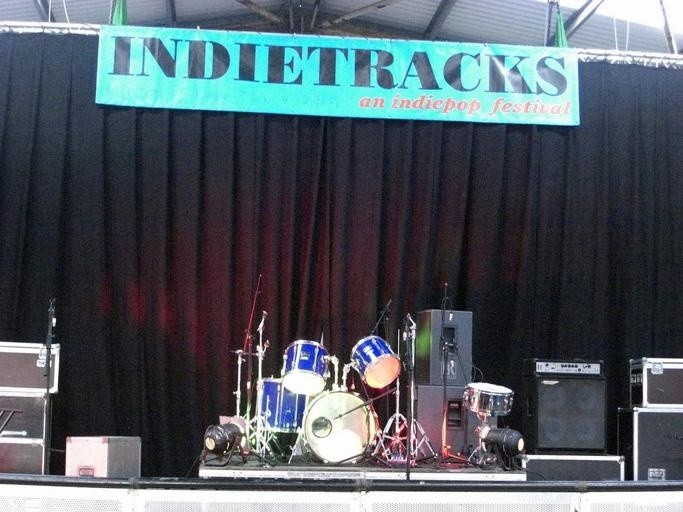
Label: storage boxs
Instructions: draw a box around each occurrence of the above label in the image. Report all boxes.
[1,339,59,474]
[618,356,682,481]
[64,434,142,479]
[522,456,627,481]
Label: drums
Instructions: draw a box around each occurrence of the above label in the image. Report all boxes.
[462,383,515,416]
[281,340,330,398]
[256,377,307,433]
[302,389,380,466]
[350,335,402,390]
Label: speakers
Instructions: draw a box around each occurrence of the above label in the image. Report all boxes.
[527,376,607,451]
[407,387,498,459]
[414,309,473,387]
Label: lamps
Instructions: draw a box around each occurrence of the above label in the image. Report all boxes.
[479,426,526,467]
[202,422,246,464]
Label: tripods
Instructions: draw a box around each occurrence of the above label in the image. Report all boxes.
[372,348,448,467]
[225,355,340,464]
[468,419,487,462]
